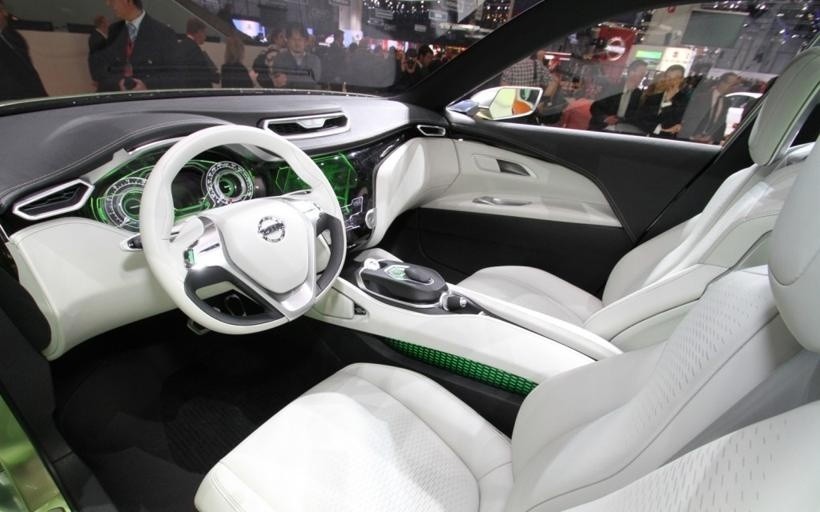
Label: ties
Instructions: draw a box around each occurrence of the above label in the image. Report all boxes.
[126,23,135,47]
[710,95,721,126]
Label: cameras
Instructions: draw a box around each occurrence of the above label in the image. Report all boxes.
[124,77,136,90]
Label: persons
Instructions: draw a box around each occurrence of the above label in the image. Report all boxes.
[255,25,457,97]
[593,61,775,144]
[104,0,188,88]
[1,1,51,100]
[86,13,115,94]
[180,18,219,88]
[219,36,252,88]
[501,52,558,123]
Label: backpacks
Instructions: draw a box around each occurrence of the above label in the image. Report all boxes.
[253,48,279,87]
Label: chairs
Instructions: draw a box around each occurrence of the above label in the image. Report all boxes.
[452,46,820,352]
[194,132,818,511]
